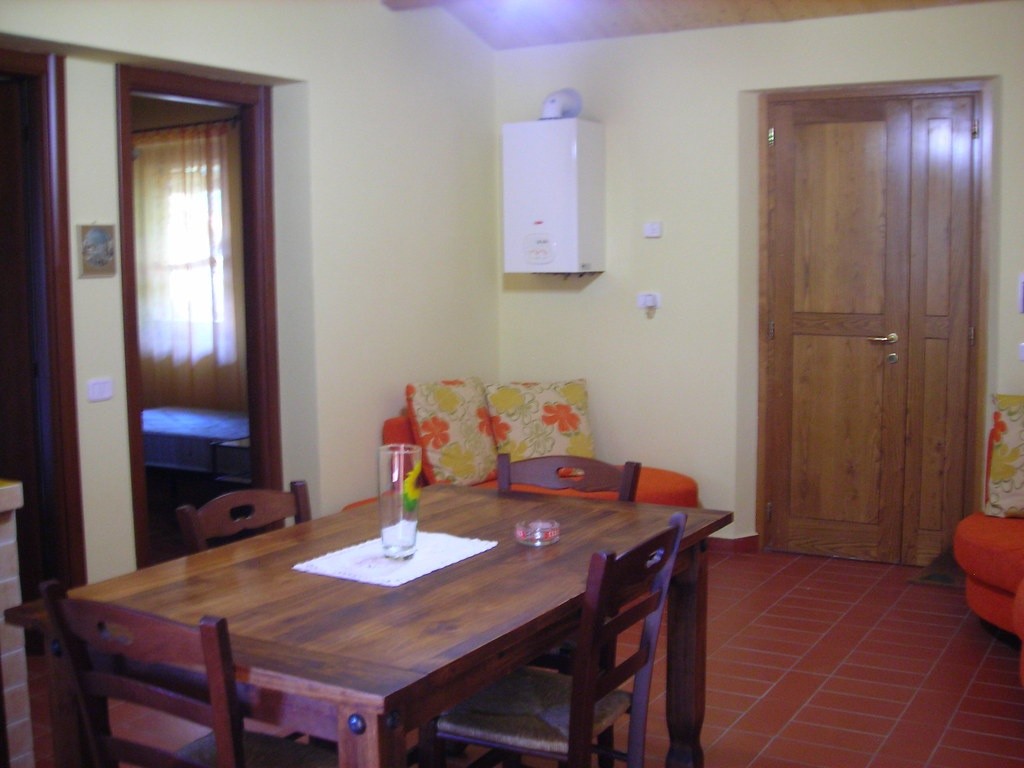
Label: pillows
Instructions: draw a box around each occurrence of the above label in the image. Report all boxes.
[406,377,500,486]
[980,391,1024,519]
[484,379,596,463]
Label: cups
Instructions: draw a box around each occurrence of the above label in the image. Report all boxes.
[378,443,423,561]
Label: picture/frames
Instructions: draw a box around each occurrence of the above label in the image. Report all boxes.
[77,223,117,279]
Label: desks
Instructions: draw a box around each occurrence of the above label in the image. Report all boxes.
[27,486,734,768]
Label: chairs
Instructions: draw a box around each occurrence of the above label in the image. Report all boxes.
[176,481,311,554]
[497,450,642,502]
[42,579,338,768]
[419,510,689,768]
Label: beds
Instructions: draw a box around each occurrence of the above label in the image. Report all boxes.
[142,406,252,476]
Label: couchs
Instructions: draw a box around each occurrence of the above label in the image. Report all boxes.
[382,407,574,491]
[953,511,1024,635]
